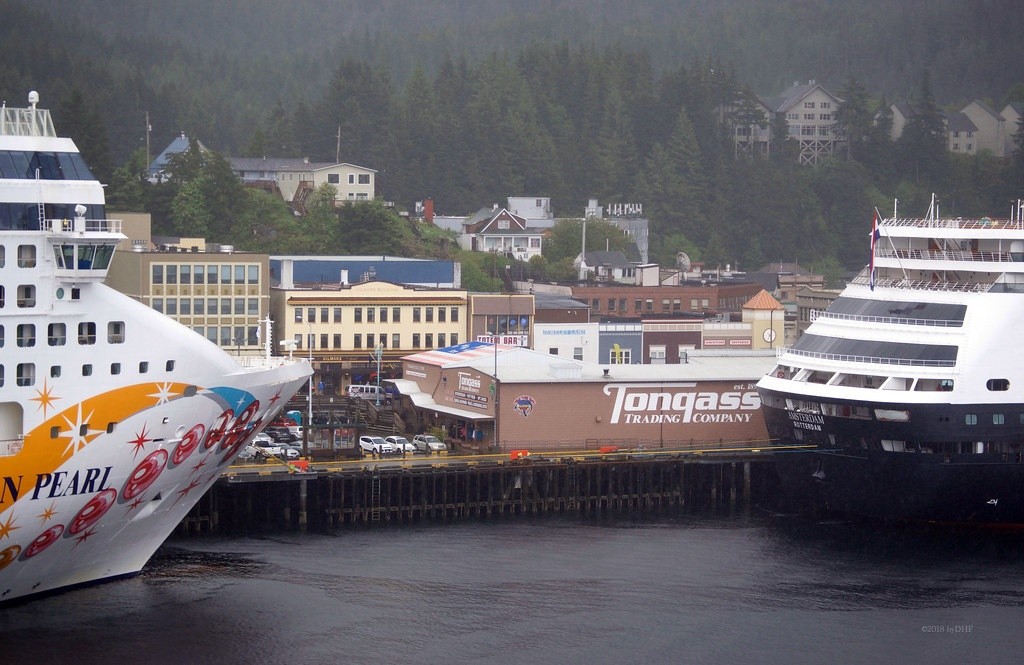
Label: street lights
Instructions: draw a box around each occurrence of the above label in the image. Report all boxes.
[296,315,312,434]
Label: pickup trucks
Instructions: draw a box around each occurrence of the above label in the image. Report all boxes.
[244,440,282,460]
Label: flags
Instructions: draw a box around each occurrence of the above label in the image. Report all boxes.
[869,213,881,291]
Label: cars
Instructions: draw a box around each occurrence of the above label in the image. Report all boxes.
[272,417,297,428]
[289,441,315,457]
[344,385,392,406]
[320,423,354,441]
[385,436,416,454]
[359,436,394,455]
[287,426,304,439]
[413,435,447,452]
[264,426,293,443]
[275,442,300,459]
[253,433,274,443]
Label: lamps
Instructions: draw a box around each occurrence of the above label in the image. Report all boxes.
[75,204,87,217]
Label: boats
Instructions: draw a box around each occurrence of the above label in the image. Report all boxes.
[756,192,1024,531]
[0,90,314,600]
